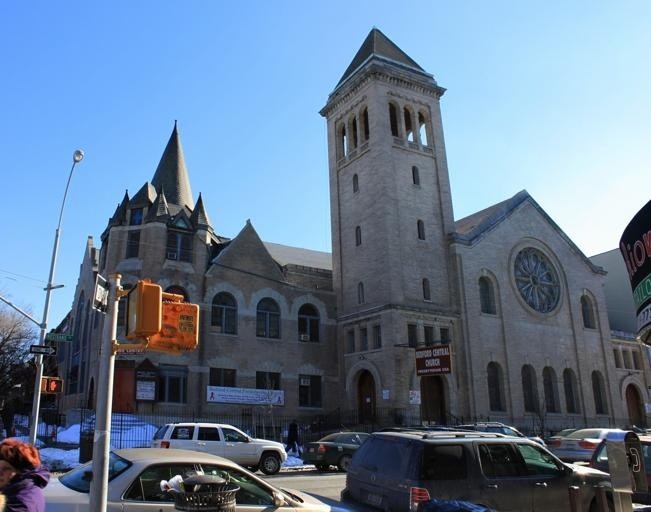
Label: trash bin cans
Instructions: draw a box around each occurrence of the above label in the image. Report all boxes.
[167,474,241,512]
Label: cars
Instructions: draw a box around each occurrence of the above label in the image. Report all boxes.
[548,427,637,463]
[301,430,370,474]
[42,448,332,512]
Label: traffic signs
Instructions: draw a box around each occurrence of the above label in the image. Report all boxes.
[47,331,75,343]
[30,344,58,356]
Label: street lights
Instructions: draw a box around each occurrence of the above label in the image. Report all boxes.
[1,145,85,447]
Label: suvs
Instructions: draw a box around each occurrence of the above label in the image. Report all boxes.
[339,431,615,512]
[458,422,547,454]
[150,422,288,476]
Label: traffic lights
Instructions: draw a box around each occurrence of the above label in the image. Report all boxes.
[127,278,163,339]
[42,374,65,395]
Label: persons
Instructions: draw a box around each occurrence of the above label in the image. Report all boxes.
[0,439,52,511]
[284,419,304,458]
